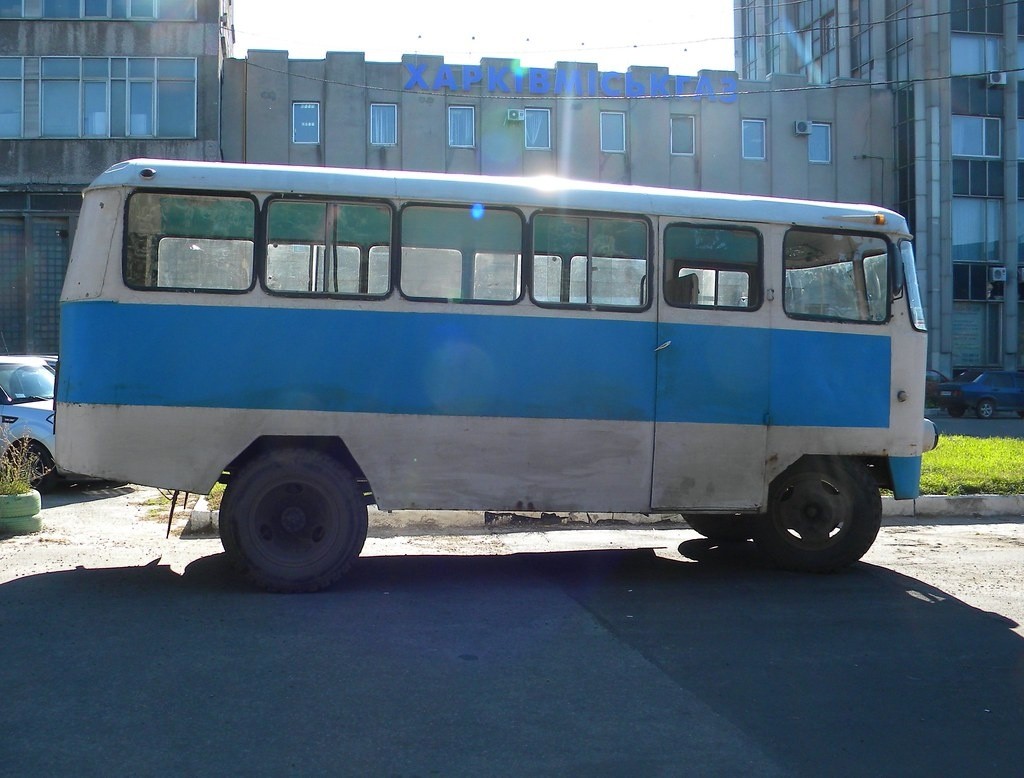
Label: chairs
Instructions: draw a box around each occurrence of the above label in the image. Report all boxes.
[668,273,702,305]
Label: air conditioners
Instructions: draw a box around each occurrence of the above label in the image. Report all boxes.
[987,70,1006,88]
[989,267,1006,282]
[794,120,813,136]
[504,108,525,121]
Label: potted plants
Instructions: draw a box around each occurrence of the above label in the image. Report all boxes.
[0,417,55,534]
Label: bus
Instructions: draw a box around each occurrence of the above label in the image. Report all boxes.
[56,157,938,595]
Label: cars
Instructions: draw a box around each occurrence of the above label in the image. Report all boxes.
[925,368,1024,419]
[0,354,106,496]
